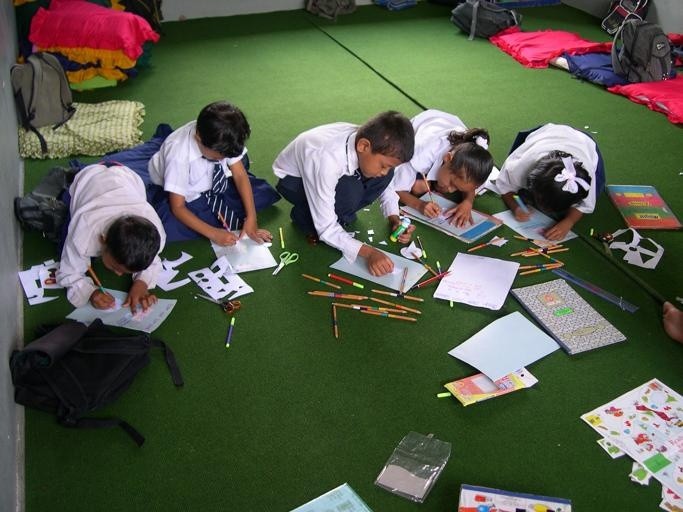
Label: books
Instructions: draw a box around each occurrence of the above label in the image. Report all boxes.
[603,184,681,231]
[508,278,627,356]
[398,191,502,244]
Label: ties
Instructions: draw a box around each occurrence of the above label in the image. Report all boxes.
[354,169,364,181]
[201,156,241,231]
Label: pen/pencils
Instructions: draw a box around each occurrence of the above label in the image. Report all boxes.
[88,264,106,294]
[300,253,454,339]
[217,210,239,243]
[416,235,426,259]
[422,172,437,213]
[226,316,235,348]
[437,391,453,398]
[279,227,285,249]
[467,237,505,251]
[513,194,529,214]
[510,234,570,275]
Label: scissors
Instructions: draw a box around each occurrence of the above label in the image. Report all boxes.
[197,293,242,313]
[272,251,299,275]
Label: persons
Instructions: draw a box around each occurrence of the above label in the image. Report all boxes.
[494,123,605,240]
[147,100,273,248]
[661,301,682,342]
[390,109,499,229]
[270,109,416,278]
[50,158,168,317]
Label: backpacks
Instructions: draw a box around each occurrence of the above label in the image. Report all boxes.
[9,320,150,422]
[11,52,72,130]
[601,0,671,83]
[451,1,522,37]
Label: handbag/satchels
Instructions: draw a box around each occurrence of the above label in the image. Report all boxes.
[16,165,77,233]
[305,1,418,20]
